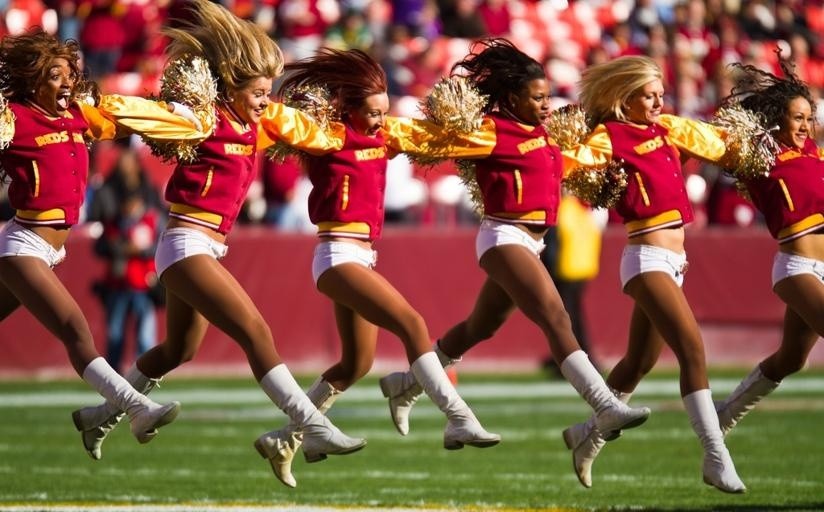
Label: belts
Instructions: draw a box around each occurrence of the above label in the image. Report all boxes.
[316,244,377,266]
[624,246,690,275]
[160,229,229,258]
[775,258,824,274]
[491,225,546,253]
[12,226,66,266]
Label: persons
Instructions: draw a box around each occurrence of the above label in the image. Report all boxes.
[96,149,161,224]
[379,36,651,442]
[368,0,650,35]
[651,0,824,61]
[71,0,368,464]
[90,195,166,376]
[1,26,181,444]
[254,48,501,489]
[715,61,824,438]
[561,54,748,495]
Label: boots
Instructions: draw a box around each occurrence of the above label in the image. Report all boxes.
[712,366,783,442]
[564,387,631,486]
[255,375,344,490]
[560,351,651,441]
[679,389,749,492]
[409,353,505,452]
[72,361,165,459]
[375,341,462,434]
[83,358,180,443]
[255,364,365,460]
